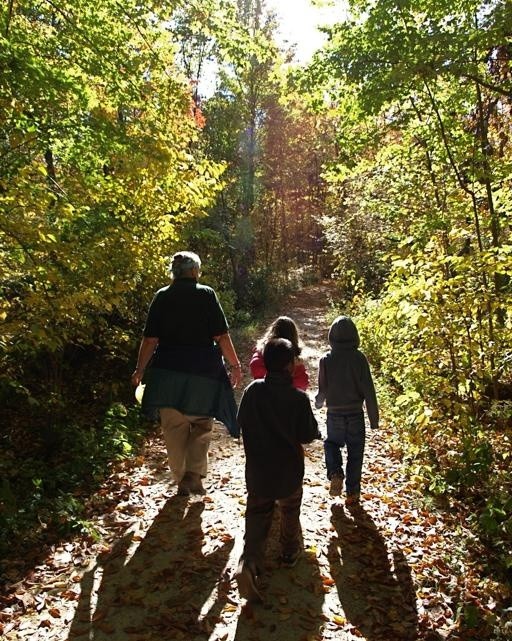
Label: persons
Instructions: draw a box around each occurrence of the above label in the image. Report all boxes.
[248,316,310,393]
[314,316,380,504]
[233,338,319,603]
[129,250,243,497]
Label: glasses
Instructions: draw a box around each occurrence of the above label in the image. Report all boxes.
[193,267,203,278]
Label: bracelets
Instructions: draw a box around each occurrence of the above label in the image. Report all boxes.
[231,361,241,368]
[135,367,145,370]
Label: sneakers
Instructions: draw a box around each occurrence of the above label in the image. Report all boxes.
[235,560,264,606]
[181,474,207,495]
[345,491,360,506]
[328,469,345,497]
[279,545,303,569]
[175,486,190,497]
